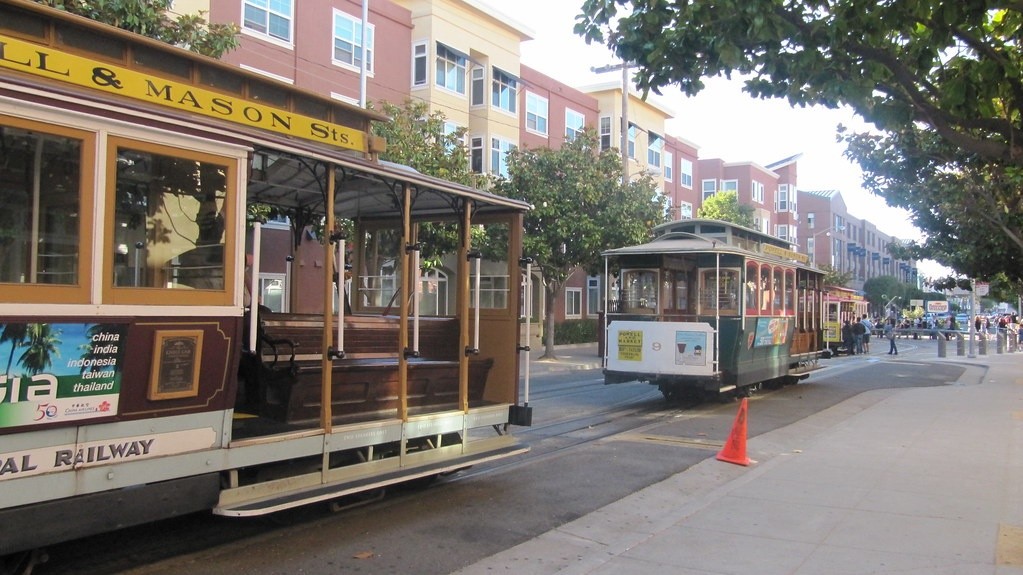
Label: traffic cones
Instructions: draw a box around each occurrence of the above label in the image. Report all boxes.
[716,396,750,465]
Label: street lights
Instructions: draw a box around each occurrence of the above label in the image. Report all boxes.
[811,225,846,269]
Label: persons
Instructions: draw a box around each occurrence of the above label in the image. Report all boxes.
[842,311,1023,356]
[177,209,282,408]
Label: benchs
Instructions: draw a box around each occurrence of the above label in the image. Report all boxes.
[242,305,495,424]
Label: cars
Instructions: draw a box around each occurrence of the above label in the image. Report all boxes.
[919,311,970,323]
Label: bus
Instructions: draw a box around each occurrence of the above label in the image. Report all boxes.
[811,280,873,355]
[598,218,830,407]
[0,0,532,574]
